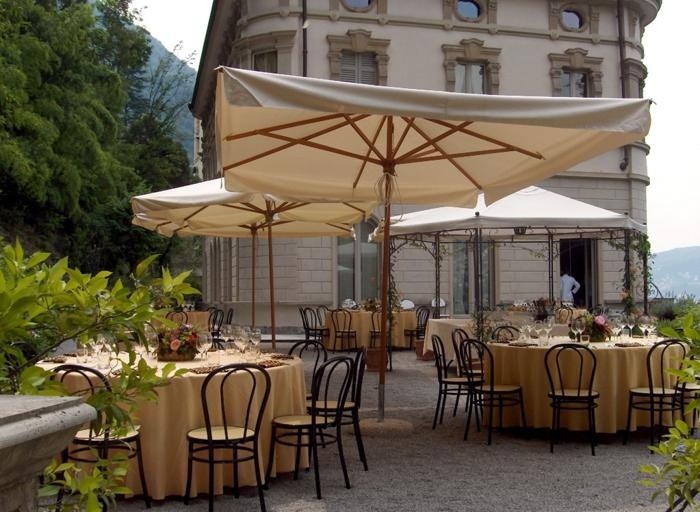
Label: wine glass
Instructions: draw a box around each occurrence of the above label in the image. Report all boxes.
[620,319,627,328]
[147,334,159,354]
[196,332,212,362]
[576,320,585,335]
[571,321,578,342]
[221,325,261,355]
[627,316,635,339]
[516,315,555,340]
[638,315,658,340]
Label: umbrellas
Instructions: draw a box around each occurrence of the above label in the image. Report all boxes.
[132,214,356,327]
[131,175,378,350]
[215,64,658,435]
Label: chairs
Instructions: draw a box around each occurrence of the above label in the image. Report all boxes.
[184,363,271,512]
[264,356,354,499]
[50,364,151,512]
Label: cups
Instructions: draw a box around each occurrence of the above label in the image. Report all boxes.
[622,329,630,340]
[541,335,550,345]
[244,345,259,363]
[580,335,589,344]
[97,351,110,368]
[74,348,88,364]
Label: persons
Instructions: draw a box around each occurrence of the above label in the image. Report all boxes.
[560,268,581,302]
